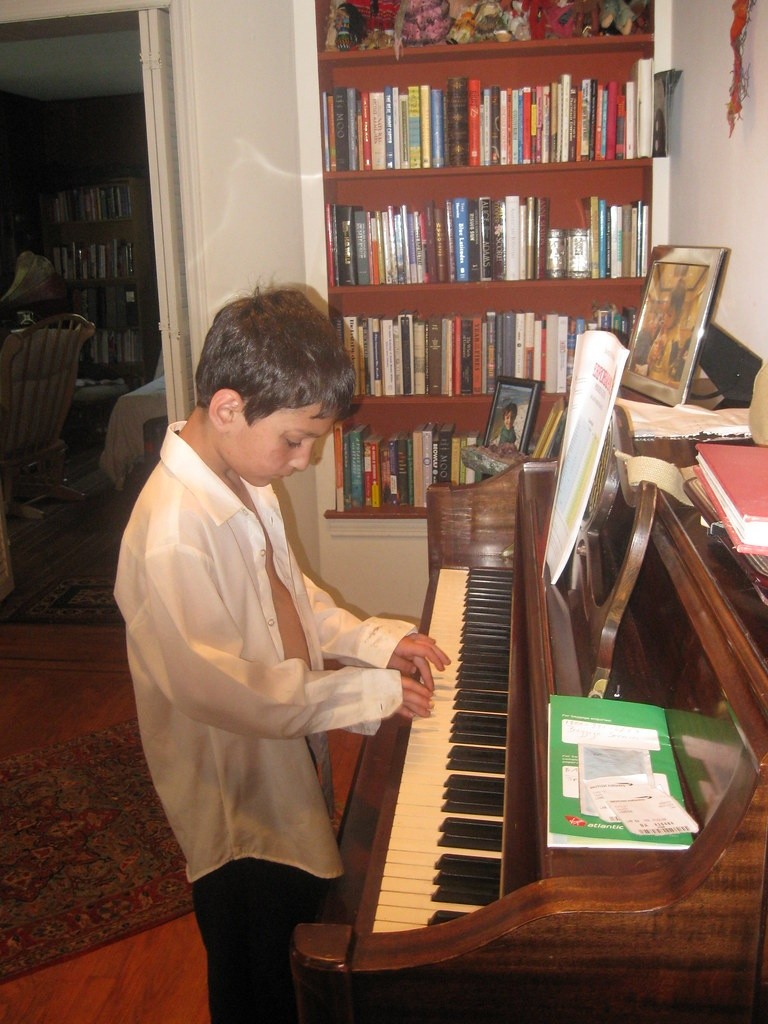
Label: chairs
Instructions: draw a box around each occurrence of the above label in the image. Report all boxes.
[0,313,95,522]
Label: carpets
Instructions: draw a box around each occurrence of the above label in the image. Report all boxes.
[0,718,342,985]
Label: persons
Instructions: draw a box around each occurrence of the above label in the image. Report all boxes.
[112,290,454,1024]
[645,285,687,385]
[498,402,517,447]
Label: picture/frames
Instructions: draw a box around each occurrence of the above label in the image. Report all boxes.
[621,246,729,404]
[483,375,545,454]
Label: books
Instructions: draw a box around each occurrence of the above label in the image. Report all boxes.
[546,692,698,850]
[682,438,768,604]
[541,330,629,588]
[333,301,640,513]
[666,705,744,828]
[324,190,649,287]
[36,179,147,381]
[320,55,686,172]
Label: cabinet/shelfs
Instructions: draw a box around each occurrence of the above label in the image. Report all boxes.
[38,176,159,387]
[323,0,654,518]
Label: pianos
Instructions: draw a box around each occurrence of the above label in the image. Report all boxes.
[288,383,768,1024]
